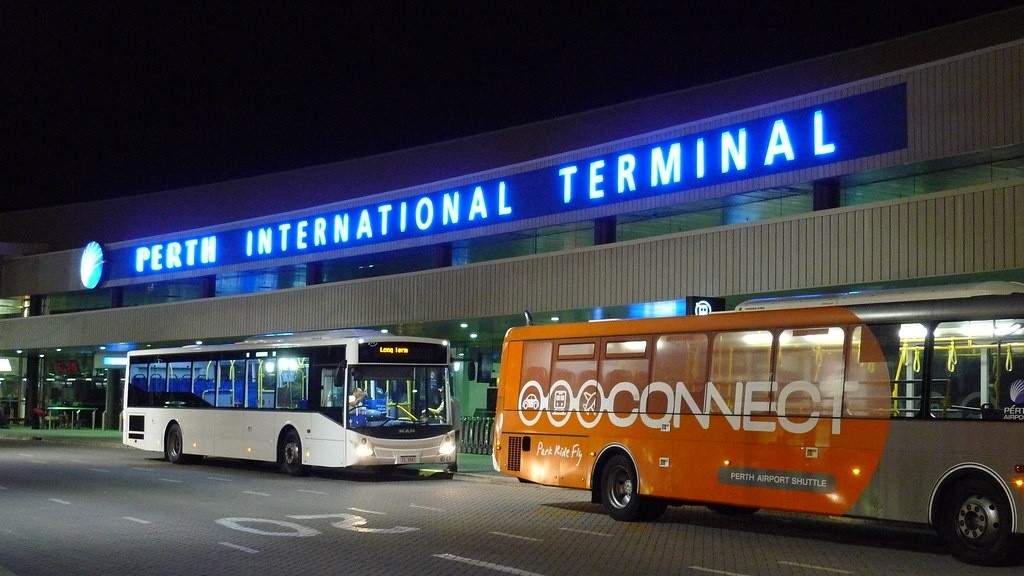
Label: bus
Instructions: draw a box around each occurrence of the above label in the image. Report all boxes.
[490,280,1024,567]
[118,328,461,477]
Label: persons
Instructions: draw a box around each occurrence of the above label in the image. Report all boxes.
[59,400,76,428]
[342,388,368,415]
[421,386,462,472]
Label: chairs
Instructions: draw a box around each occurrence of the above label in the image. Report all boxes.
[131,374,258,409]
[365,400,388,414]
